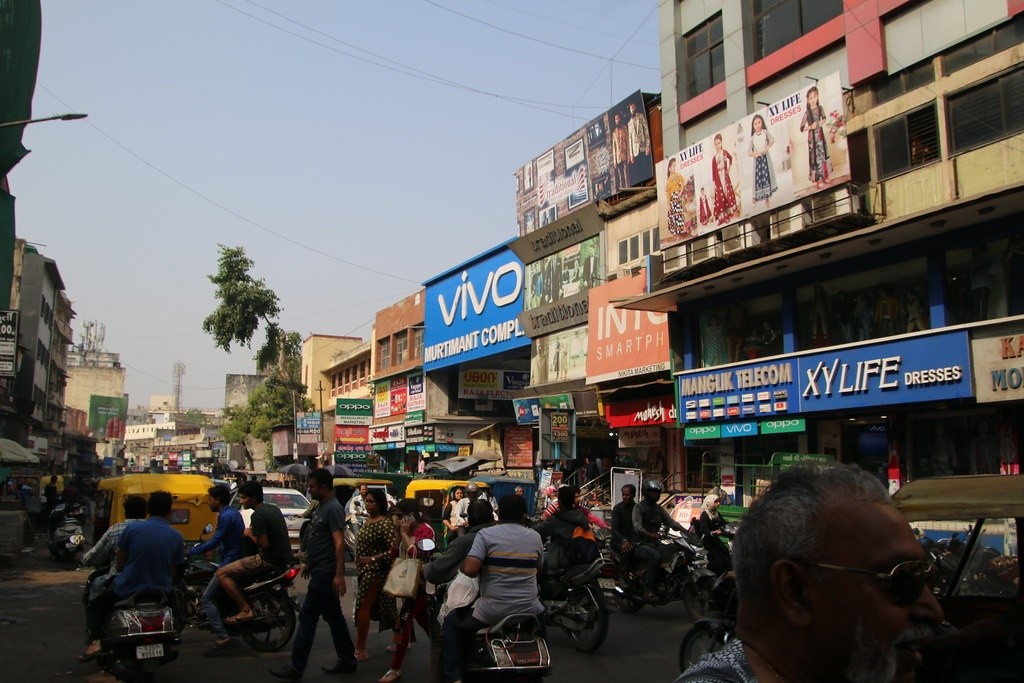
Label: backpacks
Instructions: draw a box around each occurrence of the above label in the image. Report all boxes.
[569,524,602,565]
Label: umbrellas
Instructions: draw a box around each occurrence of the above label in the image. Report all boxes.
[276,462,313,475]
[0,438,39,467]
[324,464,358,477]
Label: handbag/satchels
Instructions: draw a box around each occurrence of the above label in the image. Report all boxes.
[382,545,423,599]
[437,569,478,627]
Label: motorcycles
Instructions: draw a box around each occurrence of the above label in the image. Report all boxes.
[179,523,304,654]
[909,523,1022,598]
[30,469,543,565]
[540,537,623,655]
[608,529,724,623]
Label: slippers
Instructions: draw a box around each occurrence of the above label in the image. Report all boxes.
[226,615,254,624]
[78,650,102,665]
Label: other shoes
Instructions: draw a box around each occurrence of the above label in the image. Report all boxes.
[385,642,412,651]
[267,664,303,682]
[352,650,370,661]
[378,669,402,683]
[202,638,233,656]
[162,649,179,665]
[619,567,630,584]
[646,581,664,597]
[321,660,358,674]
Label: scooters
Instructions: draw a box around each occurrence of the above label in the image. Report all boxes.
[69,533,181,683]
[710,520,740,557]
[417,538,553,683]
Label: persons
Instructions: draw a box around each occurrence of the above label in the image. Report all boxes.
[699,494,737,577]
[612,102,651,188]
[707,487,733,528]
[712,133,738,227]
[666,158,691,238]
[748,114,778,210]
[700,187,712,225]
[530,483,610,639]
[611,484,689,603]
[125,466,296,488]
[183,481,294,656]
[44,475,59,539]
[76,489,186,665]
[443,482,524,545]
[677,459,945,683]
[800,87,833,189]
[344,482,436,683]
[61,472,111,523]
[707,477,732,506]
[562,246,597,291]
[268,467,358,679]
[531,335,568,387]
[424,494,550,683]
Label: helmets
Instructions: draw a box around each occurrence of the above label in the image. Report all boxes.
[466,484,481,501]
[641,478,661,494]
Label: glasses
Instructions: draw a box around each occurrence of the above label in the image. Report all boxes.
[792,554,946,608]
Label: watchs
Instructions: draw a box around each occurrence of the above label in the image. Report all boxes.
[372,557,375,561]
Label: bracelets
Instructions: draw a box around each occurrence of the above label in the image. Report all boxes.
[727,528,730,533]
